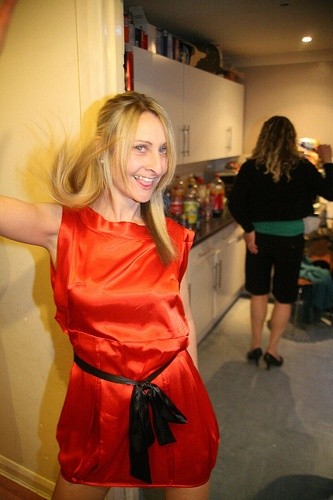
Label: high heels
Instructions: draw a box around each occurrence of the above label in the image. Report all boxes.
[264,351,284,371]
[247,348,262,366]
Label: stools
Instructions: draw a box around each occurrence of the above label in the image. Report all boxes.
[286,277,314,337]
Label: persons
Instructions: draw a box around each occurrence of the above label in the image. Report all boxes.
[229,116,333,370]
[1,6,222,498]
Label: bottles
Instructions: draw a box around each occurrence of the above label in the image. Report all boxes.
[163,173,226,231]
[298,142,320,153]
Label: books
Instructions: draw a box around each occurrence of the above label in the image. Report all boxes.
[124,12,190,64]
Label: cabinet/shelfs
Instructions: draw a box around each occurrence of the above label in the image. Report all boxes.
[125,43,245,166]
[186,220,248,344]
